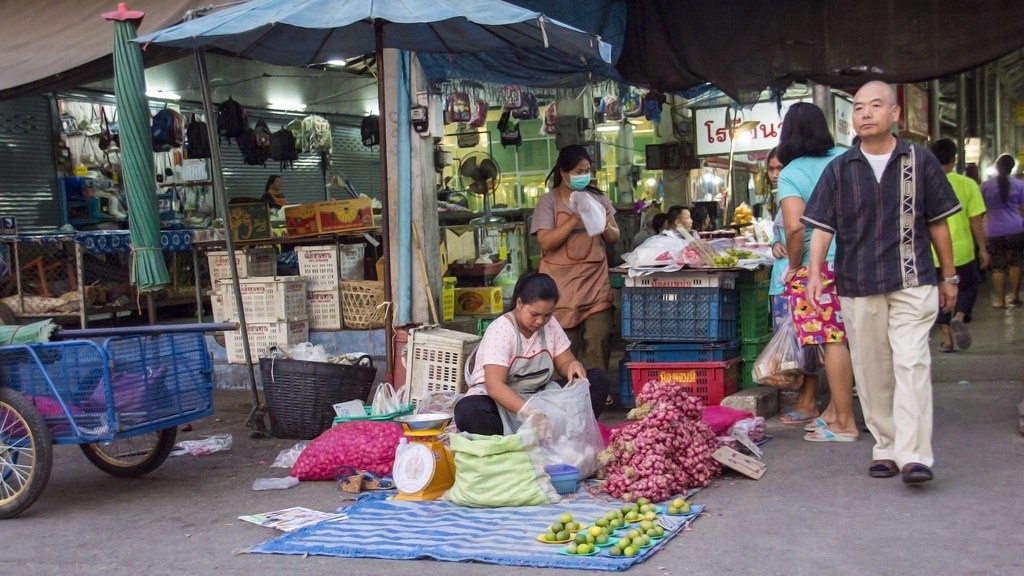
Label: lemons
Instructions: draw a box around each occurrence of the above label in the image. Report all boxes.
[735,207,753,224]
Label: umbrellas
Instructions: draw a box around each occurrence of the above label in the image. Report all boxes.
[128,0,610,389]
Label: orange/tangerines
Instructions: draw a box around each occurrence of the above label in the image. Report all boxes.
[545,497,693,555]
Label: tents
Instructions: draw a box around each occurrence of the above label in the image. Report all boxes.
[0,0,271,437]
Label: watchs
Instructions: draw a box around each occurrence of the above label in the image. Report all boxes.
[942,274,959,285]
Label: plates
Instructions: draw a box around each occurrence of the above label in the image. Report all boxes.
[536,506,698,558]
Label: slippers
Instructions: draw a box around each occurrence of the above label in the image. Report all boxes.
[950,318,972,350]
[780,410,820,425]
[803,430,857,442]
[803,418,829,429]
[938,341,954,352]
[870,460,899,477]
[902,463,933,485]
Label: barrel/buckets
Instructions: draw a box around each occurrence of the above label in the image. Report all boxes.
[493,279,518,299]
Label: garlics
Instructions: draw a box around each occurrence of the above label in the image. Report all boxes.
[589,380,766,502]
[290,424,401,480]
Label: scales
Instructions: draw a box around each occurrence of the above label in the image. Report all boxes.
[394,413,456,501]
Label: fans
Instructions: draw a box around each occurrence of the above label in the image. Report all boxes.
[458,149,504,226]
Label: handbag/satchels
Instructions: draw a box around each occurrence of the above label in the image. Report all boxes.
[63,97,127,179]
[370,381,453,417]
[752,313,826,390]
[517,377,605,482]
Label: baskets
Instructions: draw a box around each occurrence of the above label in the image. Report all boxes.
[402,322,482,411]
[259,347,378,440]
[339,281,386,328]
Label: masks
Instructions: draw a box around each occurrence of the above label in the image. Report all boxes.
[562,172,591,190]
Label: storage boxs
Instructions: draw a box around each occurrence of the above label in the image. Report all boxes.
[205,197,505,421]
[607,264,776,408]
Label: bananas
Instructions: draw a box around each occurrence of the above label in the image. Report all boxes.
[713,248,760,266]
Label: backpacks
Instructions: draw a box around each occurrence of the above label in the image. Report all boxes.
[360,115,381,148]
[150,93,334,167]
[444,82,554,148]
[598,84,666,123]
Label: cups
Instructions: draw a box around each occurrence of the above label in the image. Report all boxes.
[528,255,542,270]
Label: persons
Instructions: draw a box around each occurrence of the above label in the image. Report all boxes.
[1014,173,1024,220]
[633,207,663,249]
[530,144,619,407]
[767,147,821,424]
[930,138,990,353]
[667,205,693,231]
[454,272,610,436]
[261,175,289,210]
[653,213,668,235]
[778,102,860,441]
[965,164,989,299]
[979,154,1024,308]
[689,206,710,238]
[799,80,963,483]
